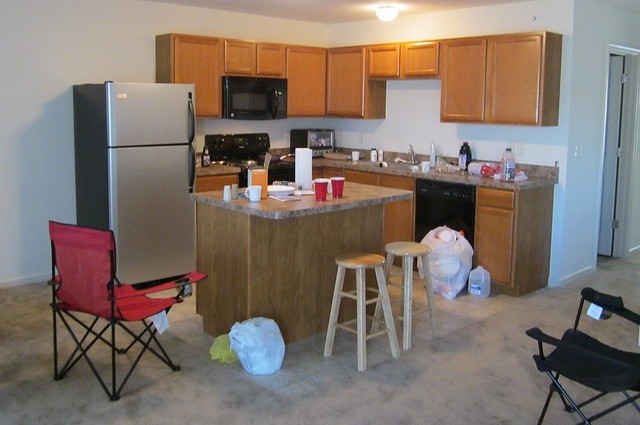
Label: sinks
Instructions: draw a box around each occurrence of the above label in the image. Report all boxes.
[361,160,421,173]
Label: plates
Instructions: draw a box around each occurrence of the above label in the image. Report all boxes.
[267,185,295,196]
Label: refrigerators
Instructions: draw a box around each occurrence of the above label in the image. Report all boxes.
[72,83,197,286]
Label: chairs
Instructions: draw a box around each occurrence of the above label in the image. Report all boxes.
[49,221,207,401]
[525,287,640,425]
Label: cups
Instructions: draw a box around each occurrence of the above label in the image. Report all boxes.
[352,151,360,160]
[245,185,262,202]
[331,177,345,199]
[314,180,328,201]
[421,161,431,173]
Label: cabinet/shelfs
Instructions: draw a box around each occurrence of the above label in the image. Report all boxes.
[345,172,415,242]
[439,36,560,128]
[367,43,441,80]
[288,47,329,116]
[224,39,287,78]
[475,189,515,282]
[325,45,367,119]
[173,34,223,119]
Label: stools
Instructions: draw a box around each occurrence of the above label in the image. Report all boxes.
[370,240,439,350]
[324,253,401,370]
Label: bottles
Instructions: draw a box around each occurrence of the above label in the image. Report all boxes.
[370,148,378,162]
[500,148,515,183]
[202,146,211,167]
[459,142,471,171]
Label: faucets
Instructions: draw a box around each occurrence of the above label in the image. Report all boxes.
[408,144,418,165]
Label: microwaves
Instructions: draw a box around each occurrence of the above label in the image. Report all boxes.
[290,128,335,157]
[221,75,288,121]
